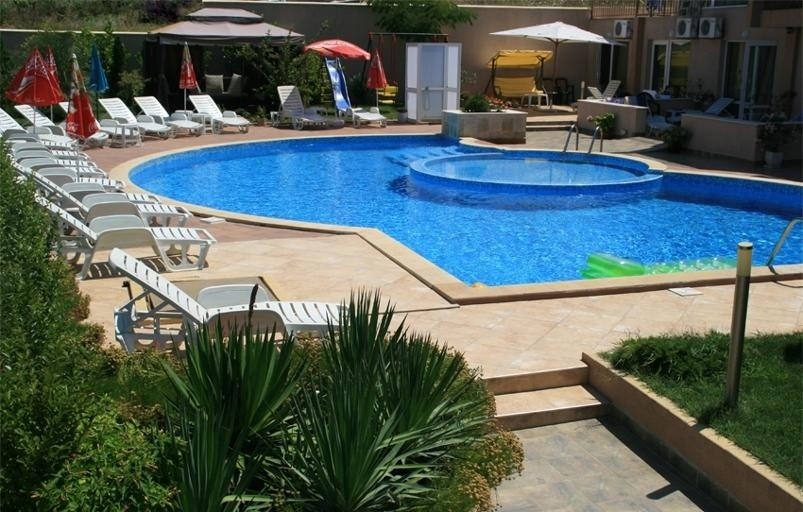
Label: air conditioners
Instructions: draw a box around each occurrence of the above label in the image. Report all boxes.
[675,17,697,38]
[613,19,633,39]
[699,16,723,38]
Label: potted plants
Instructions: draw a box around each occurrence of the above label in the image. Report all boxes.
[758,114,790,170]
[659,125,693,153]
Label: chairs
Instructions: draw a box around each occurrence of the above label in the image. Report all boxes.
[269,85,329,130]
[339,107,388,128]
[542,77,734,139]
[107,247,357,355]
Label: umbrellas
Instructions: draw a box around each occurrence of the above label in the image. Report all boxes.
[65,51,101,184]
[302,37,371,115]
[178,41,199,110]
[148,5,305,49]
[42,44,61,125]
[6,46,68,135]
[365,46,389,110]
[89,43,110,123]
[486,17,631,112]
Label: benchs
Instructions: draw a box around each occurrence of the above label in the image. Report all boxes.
[495,76,543,107]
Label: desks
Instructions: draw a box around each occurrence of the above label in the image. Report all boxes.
[520,93,549,108]
